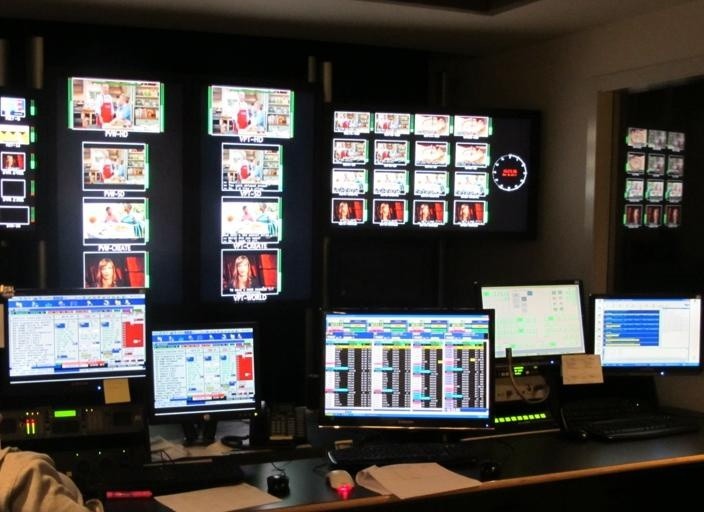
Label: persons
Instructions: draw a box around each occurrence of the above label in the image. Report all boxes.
[4,155,16,169]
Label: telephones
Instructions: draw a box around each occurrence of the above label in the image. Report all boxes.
[249,400,307,449]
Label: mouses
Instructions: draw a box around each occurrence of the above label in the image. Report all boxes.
[573,424,591,443]
[266,473,289,497]
[480,460,501,481]
[328,468,355,492]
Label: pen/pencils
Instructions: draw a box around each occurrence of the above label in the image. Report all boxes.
[106,490,153,499]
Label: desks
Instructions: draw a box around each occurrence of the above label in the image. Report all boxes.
[0,401,704,511]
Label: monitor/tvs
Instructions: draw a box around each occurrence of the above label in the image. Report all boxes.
[623,125,686,231]
[593,293,703,375]
[147,324,257,448]
[322,103,544,242]
[316,306,496,431]
[42,66,323,313]
[3,288,151,406]
[472,278,586,359]
[1,84,51,241]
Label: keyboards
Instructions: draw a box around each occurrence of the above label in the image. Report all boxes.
[587,409,698,441]
[326,438,473,471]
